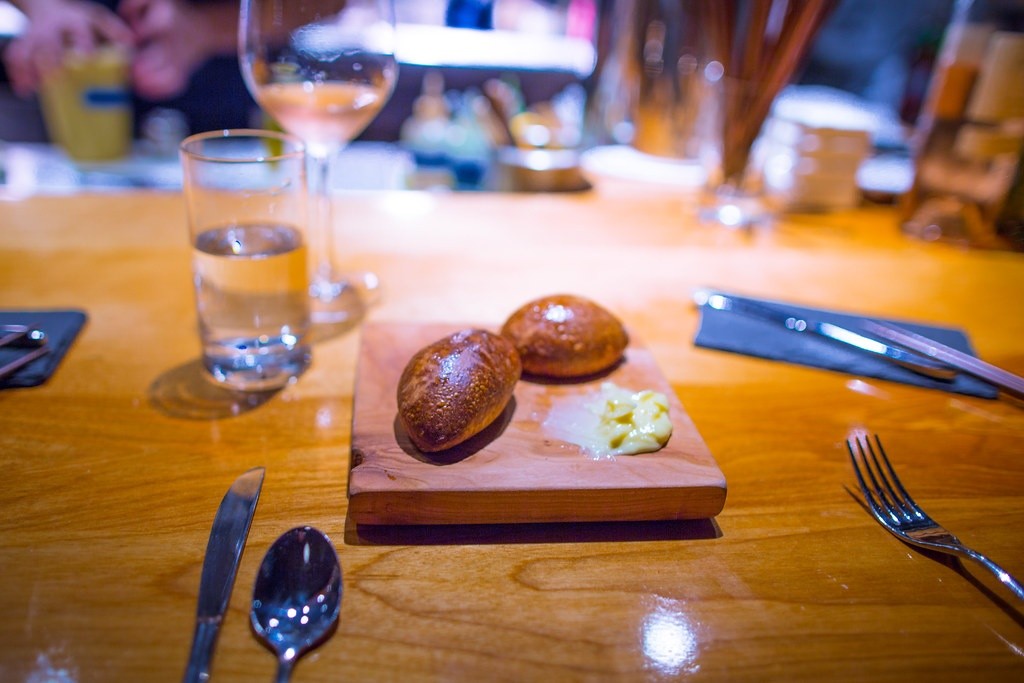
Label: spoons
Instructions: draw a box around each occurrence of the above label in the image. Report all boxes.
[251,526,343,682]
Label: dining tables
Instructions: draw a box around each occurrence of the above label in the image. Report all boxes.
[0,187,1024,683]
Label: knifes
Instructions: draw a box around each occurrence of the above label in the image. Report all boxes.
[690,285,955,382]
[181,465,266,683]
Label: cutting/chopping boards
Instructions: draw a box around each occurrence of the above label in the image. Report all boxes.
[349,321,727,525]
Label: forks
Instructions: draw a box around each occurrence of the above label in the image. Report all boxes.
[845,435,1024,602]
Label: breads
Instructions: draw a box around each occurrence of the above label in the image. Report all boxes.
[397,327,523,452]
[499,295,628,379]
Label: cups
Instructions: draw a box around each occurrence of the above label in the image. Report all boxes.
[760,97,876,211]
[180,129,313,388]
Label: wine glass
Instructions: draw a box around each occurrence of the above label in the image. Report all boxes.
[237,1,398,319]
